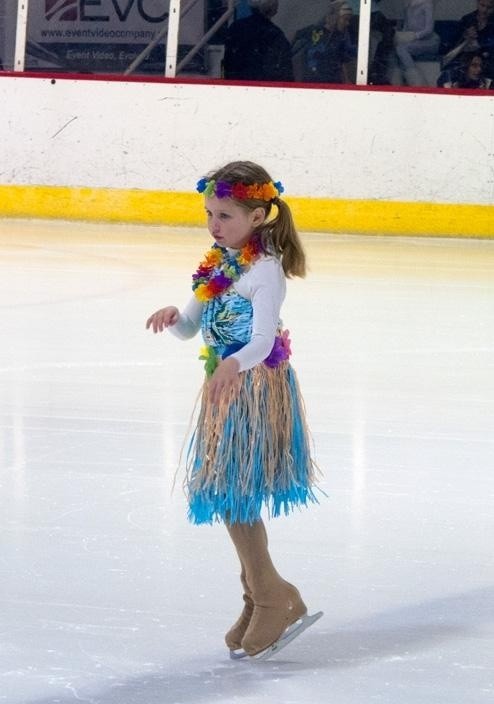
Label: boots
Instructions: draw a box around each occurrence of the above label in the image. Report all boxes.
[224,508,308,657]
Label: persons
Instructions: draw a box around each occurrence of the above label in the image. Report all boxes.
[146,160,328,656]
[222,1,493,87]
